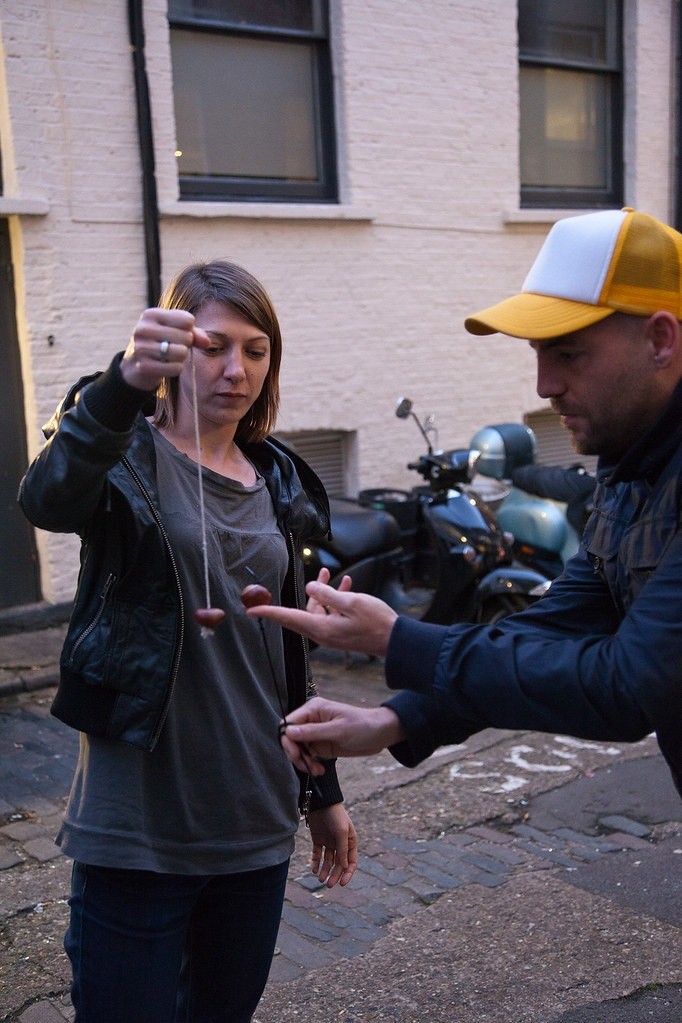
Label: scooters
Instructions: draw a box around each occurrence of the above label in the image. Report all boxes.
[310,393,589,654]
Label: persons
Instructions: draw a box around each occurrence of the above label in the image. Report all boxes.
[246,207,682,800]
[17,260,358,1023]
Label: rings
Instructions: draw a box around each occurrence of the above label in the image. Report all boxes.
[160,340,170,359]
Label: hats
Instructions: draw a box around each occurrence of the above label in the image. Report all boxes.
[464,206,682,339]
